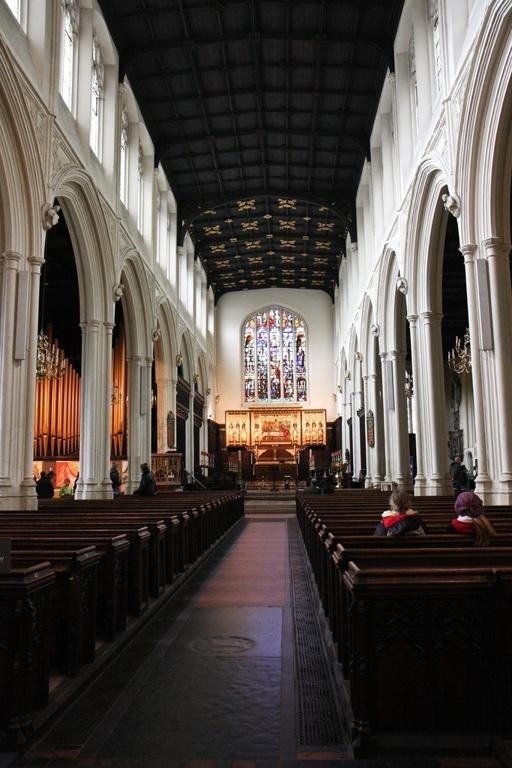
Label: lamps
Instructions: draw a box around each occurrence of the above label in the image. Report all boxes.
[37,264,66,380]
[448,327,471,375]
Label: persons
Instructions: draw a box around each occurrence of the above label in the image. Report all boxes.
[213,464,233,490]
[460,465,478,492]
[302,470,366,495]
[449,456,465,494]
[33,462,121,499]
[373,488,428,536]
[133,462,155,495]
[229,418,323,444]
[445,491,497,546]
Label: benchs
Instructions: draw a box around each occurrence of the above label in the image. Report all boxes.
[0,490,244,753]
[295,491,512,758]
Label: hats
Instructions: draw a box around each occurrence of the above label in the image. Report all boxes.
[455,492,485,518]
